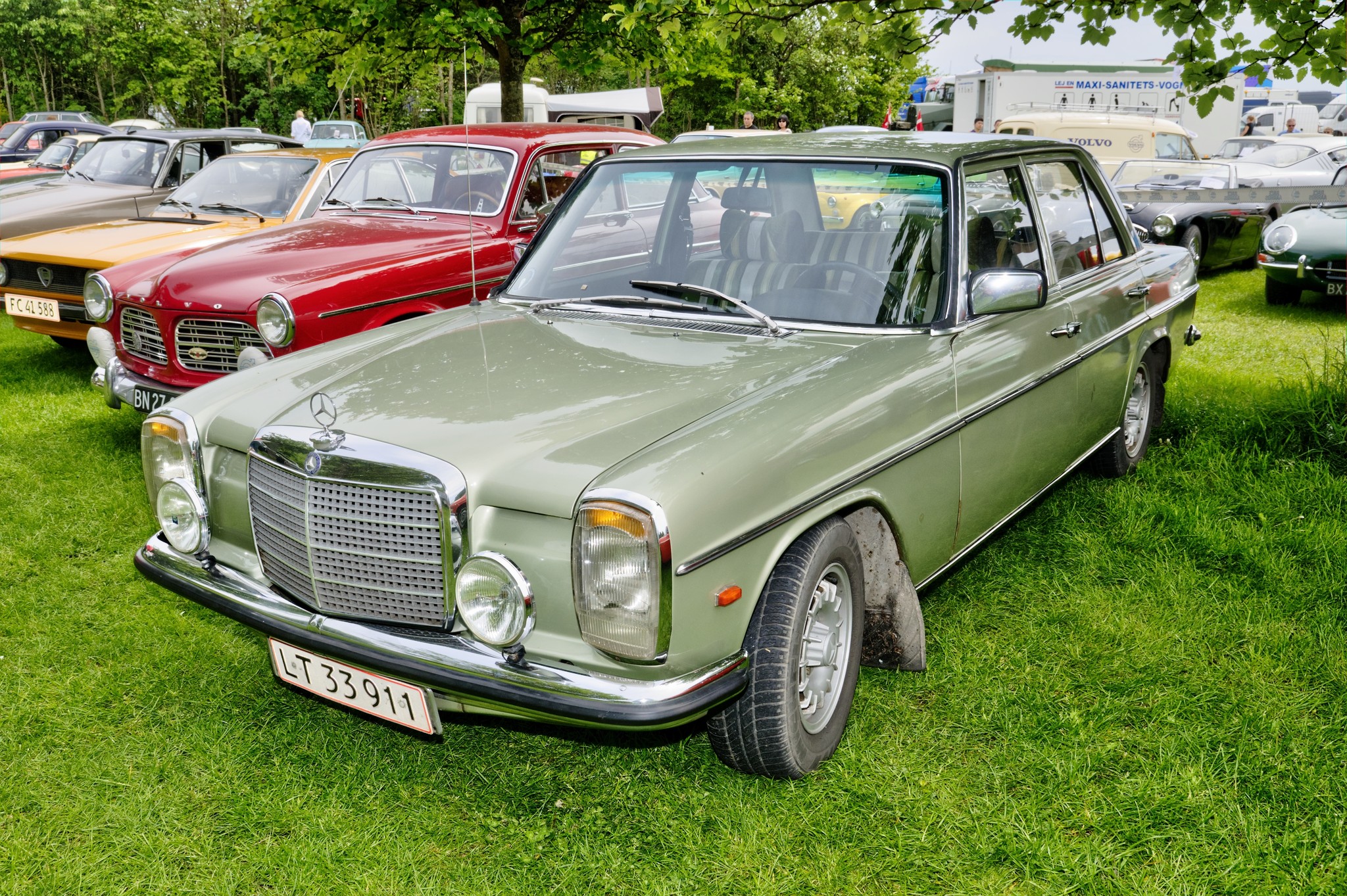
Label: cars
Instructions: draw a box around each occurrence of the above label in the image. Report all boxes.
[0,89,1347,781]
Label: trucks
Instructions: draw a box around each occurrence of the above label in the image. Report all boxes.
[952,68,1245,159]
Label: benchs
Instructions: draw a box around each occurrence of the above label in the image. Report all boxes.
[800,202,1013,298]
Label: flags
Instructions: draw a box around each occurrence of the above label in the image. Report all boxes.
[914,109,924,131]
[881,103,892,130]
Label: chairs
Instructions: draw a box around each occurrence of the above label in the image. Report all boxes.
[869,216,980,330]
[682,207,826,323]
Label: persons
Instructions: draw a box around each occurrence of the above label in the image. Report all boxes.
[1240,115,1256,136]
[147,99,183,128]
[1323,127,1335,135]
[290,110,312,143]
[990,119,1003,133]
[41,116,66,150]
[739,111,759,130]
[1262,118,1303,147]
[776,114,792,134]
[969,117,984,133]
